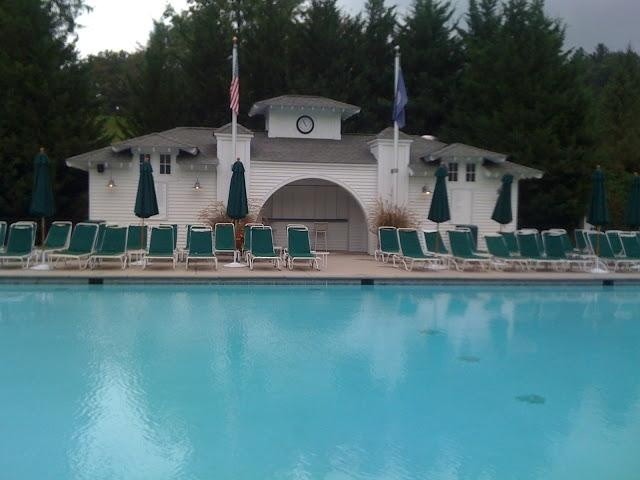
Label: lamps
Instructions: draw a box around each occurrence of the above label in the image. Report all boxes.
[193,172,203,191]
[422,179,431,195]
[106,162,118,188]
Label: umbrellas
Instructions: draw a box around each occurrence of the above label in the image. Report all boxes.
[624,172,640,231]
[131,152,162,260]
[585,164,611,258]
[491,173,515,248]
[428,160,451,259]
[226,156,251,261]
[28,143,60,262]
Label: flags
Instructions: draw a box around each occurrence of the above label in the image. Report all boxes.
[391,55,411,129]
[228,43,242,121]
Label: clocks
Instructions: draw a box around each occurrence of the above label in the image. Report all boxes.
[296,115,315,135]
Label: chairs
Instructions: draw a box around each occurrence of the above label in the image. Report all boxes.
[390,287,639,322]
[375,225,640,274]
